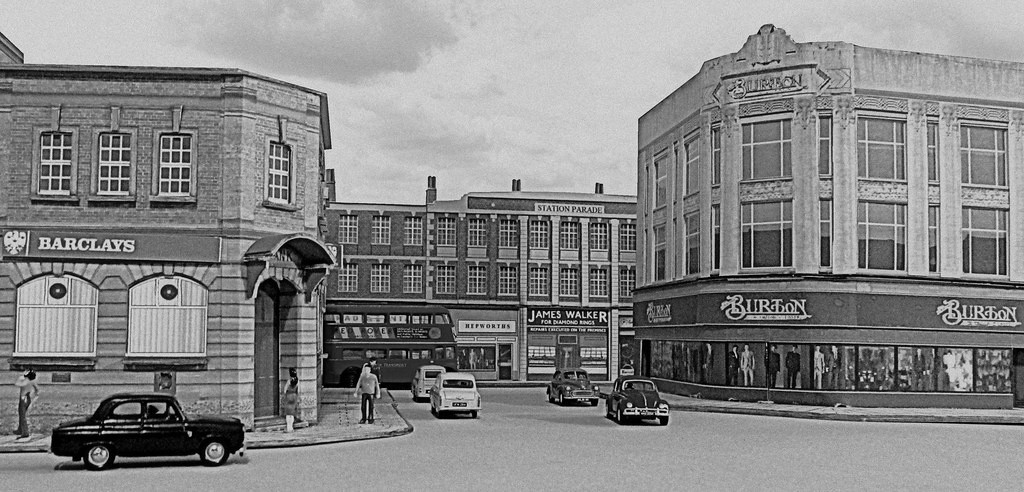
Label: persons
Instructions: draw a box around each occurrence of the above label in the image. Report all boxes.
[477,347,487,368]
[741,344,755,386]
[786,345,801,388]
[461,349,469,369]
[354,365,381,424]
[825,345,842,390]
[12,369,40,439]
[813,344,824,390]
[769,344,780,387]
[673,341,714,384]
[367,356,381,399]
[469,349,478,368]
[898,354,951,393]
[728,344,740,385]
[283,367,300,433]
[847,350,889,390]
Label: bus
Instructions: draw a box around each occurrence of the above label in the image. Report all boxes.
[322,303,461,388]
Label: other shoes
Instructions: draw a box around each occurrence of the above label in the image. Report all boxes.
[368,418,374,424]
[358,418,365,424]
[17,434,29,439]
[13,429,21,434]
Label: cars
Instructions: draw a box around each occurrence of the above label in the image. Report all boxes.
[605,374,670,426]
[547,367,600,406]
[50,391,246,471]
[426,371,483,419]
[411,364,446,403]
